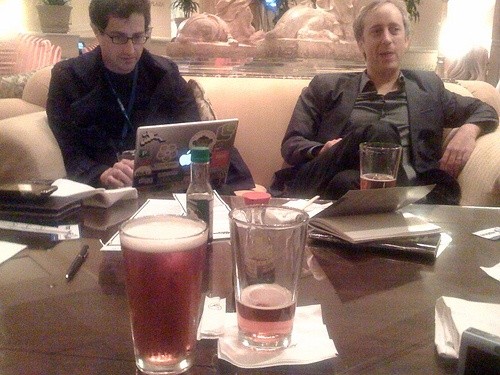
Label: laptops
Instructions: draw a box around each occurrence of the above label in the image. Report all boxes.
[117,118,240,194]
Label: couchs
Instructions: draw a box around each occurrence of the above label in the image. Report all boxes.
[0,64,499,207]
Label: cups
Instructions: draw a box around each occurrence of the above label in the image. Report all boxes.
[229,204,309,351]
[359,142,403,189]
[119,214,209,375]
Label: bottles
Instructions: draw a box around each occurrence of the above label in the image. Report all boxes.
[242,191,277,284]
[186,146,214,246]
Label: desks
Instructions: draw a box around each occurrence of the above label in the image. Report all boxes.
[0,194,500,374]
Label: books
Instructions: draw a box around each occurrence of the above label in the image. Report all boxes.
[308,183,452,260]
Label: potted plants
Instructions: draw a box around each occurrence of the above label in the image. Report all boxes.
[171,0,200,28]
[35,1,73,34]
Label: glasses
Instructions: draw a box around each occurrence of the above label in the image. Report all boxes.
[92,22,148,44]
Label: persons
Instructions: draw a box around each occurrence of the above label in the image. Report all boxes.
[46,0,256,195]
[267,1,499,205]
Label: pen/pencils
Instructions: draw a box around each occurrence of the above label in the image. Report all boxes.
[65,244,89,279]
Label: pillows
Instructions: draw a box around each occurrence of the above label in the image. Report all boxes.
[0,67,42,99]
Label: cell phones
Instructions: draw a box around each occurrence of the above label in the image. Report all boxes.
[1,184,59,203]
